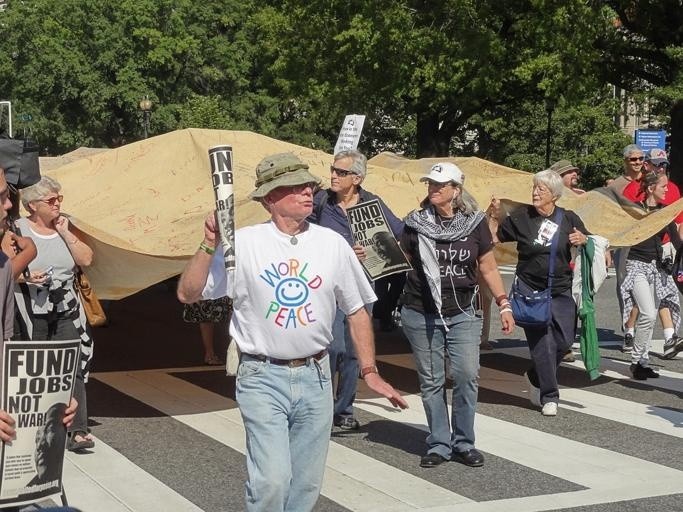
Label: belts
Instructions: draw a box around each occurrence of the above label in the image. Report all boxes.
[248,350,327,367]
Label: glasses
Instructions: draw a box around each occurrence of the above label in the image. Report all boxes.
[331,166,357,176]
[629,156,643,161]
[40,195,63,205]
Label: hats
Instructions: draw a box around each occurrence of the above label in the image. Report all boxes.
[551,160,578,175]
[420,162,464,184]
[249,153,321,201]
[645,149,671,167]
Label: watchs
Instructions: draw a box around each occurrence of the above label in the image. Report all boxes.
[357,365,378,379]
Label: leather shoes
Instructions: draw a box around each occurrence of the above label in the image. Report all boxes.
[457,449,483,466]
[421,453,445,467]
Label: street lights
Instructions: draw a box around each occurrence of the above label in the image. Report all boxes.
[544,96,558,171]
[138,94,154,139]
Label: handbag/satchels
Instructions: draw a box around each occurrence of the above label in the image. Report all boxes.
[508,275,552,328]
[73,271,106,326]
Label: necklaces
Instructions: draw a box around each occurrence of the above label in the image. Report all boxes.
[288,219,307,245]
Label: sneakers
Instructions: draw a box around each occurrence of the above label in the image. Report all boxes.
[622,333,635,353]
[663,334,683,359]
[525,371,541,407]
[334,416,358,430]
[561,349,574,362]
[630,362,659,380]
[67,432,94,451]
[542,402,558,415]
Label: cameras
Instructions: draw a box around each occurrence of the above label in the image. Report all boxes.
[662,258,674,276]
[42,266,54,285]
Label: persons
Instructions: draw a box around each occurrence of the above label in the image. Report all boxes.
[487,169,610,417]
[0,164,96,511]
[374,272,406,330]
[220,194,234,257]
[549,143,683,381]
[182,296,233,365]
[478,271,494,350]
[371,231,407,269]
[307,148,405,433]
[397,160,515,468]
[174,147,411,511]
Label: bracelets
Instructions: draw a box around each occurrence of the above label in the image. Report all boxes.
[498,301,513,314]
[489,213,499,221]
[496,294,509,307]
[199,241,216,255]
